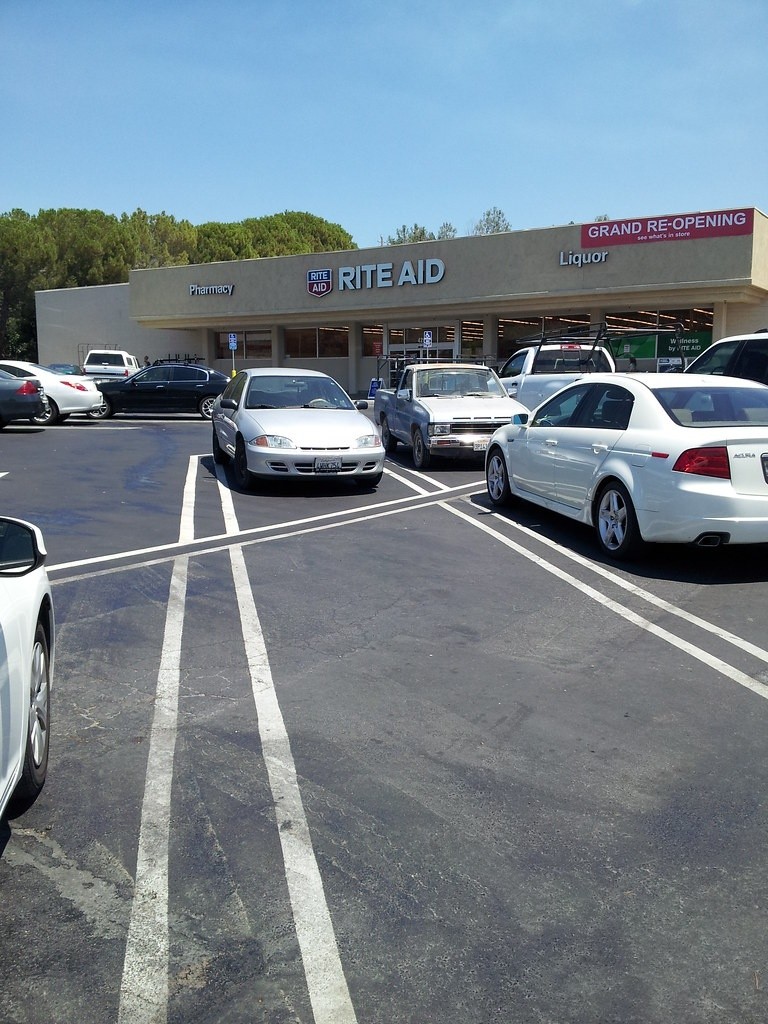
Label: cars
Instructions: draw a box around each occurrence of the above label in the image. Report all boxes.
[212,368,385,489]
[484,373,767,558]
[0,370,49,433]
[0,515,57,844]
[49,363,85,378]
[87,363,234,421]
[0,360,104,425]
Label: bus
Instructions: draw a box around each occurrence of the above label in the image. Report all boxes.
[602,328,713,375]
[82,349,142,385]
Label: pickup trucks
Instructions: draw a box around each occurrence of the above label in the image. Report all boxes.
[487,321,618,415]
[374,363,533,471]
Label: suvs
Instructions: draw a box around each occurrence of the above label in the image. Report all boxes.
[682,328,768,393]
